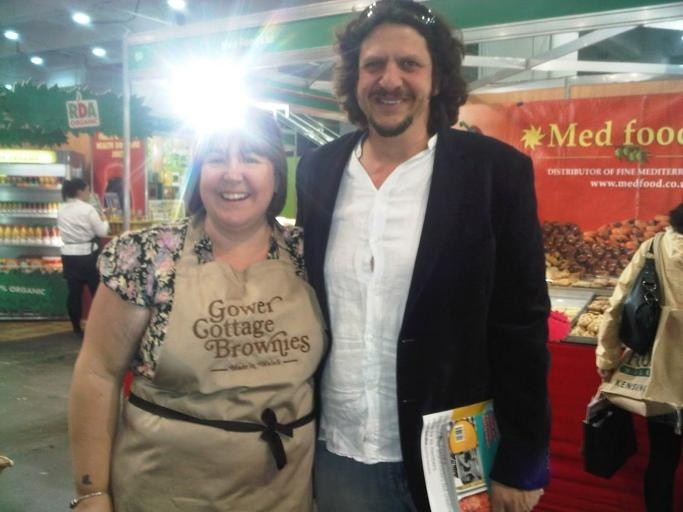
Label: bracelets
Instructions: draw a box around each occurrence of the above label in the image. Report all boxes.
[69,489,112,507]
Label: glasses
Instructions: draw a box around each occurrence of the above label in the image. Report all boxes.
[357,1,437,34]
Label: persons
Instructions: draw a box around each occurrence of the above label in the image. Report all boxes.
[594,202,681,512]
[289,1,552,512]
[66,107,331,512]
[58,178,110,335]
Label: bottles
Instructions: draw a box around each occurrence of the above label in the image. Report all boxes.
[0,201,59,214]
[0,175,63,188]
[0,223,61,245]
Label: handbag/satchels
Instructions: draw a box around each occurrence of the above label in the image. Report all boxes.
[619,259,662,355]
[583,406,637,478]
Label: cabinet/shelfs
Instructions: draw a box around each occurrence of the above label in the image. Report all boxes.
[0,145,85,325]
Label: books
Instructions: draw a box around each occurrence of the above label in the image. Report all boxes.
[415,397,494,511]
